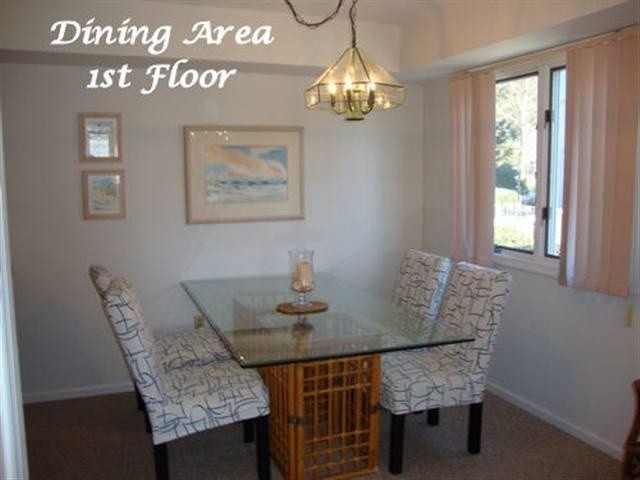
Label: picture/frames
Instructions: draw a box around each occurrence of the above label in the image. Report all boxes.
[78,111,127,220]
[181,122,307,225]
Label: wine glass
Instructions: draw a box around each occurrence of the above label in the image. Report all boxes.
[287,246,318,309]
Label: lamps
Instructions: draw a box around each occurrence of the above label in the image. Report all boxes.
[304,0,405,126]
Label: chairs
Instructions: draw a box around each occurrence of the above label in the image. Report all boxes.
[89,250,513,480]
